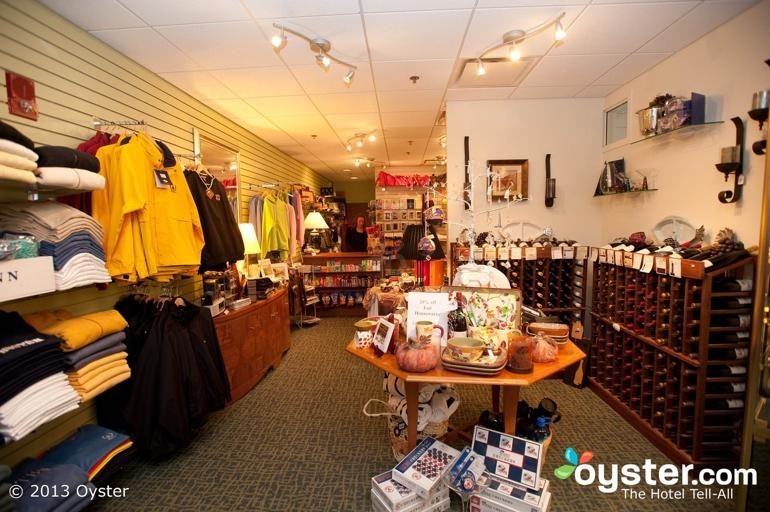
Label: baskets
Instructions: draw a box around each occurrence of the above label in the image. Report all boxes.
[363,397,450,462]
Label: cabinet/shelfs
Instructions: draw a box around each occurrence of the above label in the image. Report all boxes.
[210,285,292,408]
[296,183,346,253]
[452,242,758,479]
[0,176,128,512]
[298,251,384,317]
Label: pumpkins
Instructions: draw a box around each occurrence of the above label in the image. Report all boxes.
[396,340,439,373]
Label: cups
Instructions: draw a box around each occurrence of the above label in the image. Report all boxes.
[416,322,443,337]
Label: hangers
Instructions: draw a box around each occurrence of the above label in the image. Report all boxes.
[175,152,214,179]
[257,183,289,200]
[126,279,188,312]
[95,119,147,140]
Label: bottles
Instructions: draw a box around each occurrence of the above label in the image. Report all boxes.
[601,162,618,194]
[447,235,752,450]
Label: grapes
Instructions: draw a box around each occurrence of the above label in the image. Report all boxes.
[710,238,741,258]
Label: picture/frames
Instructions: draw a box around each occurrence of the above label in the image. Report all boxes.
[376,198,422,232]
[372,317,396,354]
[487,159,528,205]
[440,286,522,342]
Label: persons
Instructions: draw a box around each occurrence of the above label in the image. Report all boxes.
[346,215,368,252]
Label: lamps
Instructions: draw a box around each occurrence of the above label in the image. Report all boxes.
[270,19,358,86]
[424,157,447,169]
[472,11,570,77]
[464,136,471,210]
[546,154,557,207]
[714,116,744,204]
[346,133,376,152]
[238,223,262,278]
[748,89,769,154]
[304,209,329,254]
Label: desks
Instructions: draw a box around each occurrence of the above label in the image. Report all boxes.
[345,330,588,454]
